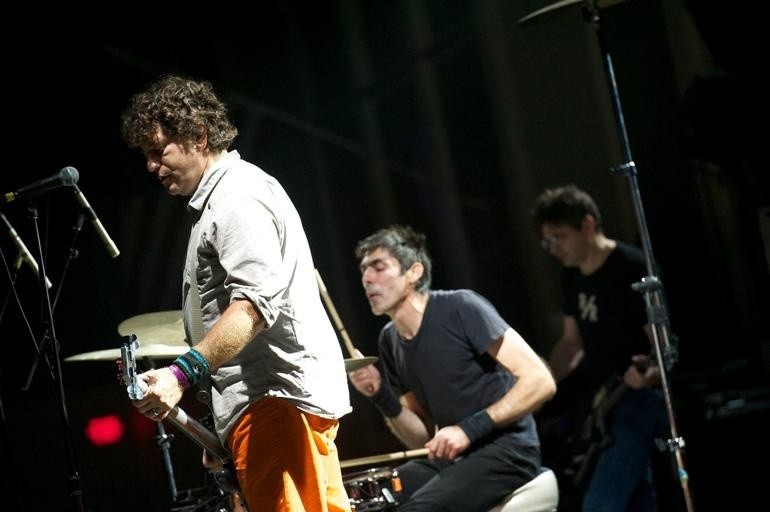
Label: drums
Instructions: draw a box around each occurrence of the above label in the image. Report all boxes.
[343,466,403,512]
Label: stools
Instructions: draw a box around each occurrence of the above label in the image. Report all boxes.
[481,466,561,512]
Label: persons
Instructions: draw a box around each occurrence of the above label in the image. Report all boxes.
[122,74,353,512]
[348,223,557,512]
[531,186,678,512]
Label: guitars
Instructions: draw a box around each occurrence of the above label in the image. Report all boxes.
[119,339,250,512]
[557,325,678,483]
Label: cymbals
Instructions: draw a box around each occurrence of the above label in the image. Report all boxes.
[343,356,378,371]
[66,342,192,360]
[117,308,188,342]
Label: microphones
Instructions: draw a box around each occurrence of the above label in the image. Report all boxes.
[4,166,78,202]
[69,185,120,259]
[0,214,53,290]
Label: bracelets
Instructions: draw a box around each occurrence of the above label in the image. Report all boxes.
[457,409,497,445]
[170,364,190,391]
[174,349,212,386]
[366,378,402,418]
